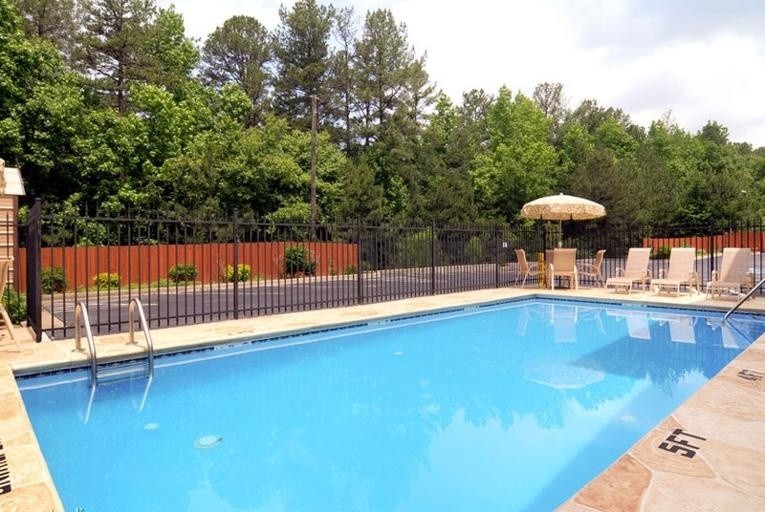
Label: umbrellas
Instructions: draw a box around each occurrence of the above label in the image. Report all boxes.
[526,351,607,387]
[521,193,606,248]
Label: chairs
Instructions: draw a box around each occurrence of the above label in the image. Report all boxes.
[516,304,754,349]
[514,247,606,290]
[651,247,700,298]
[706,247,753,303]
[605,247,653,296]
[0,260,22,353]
